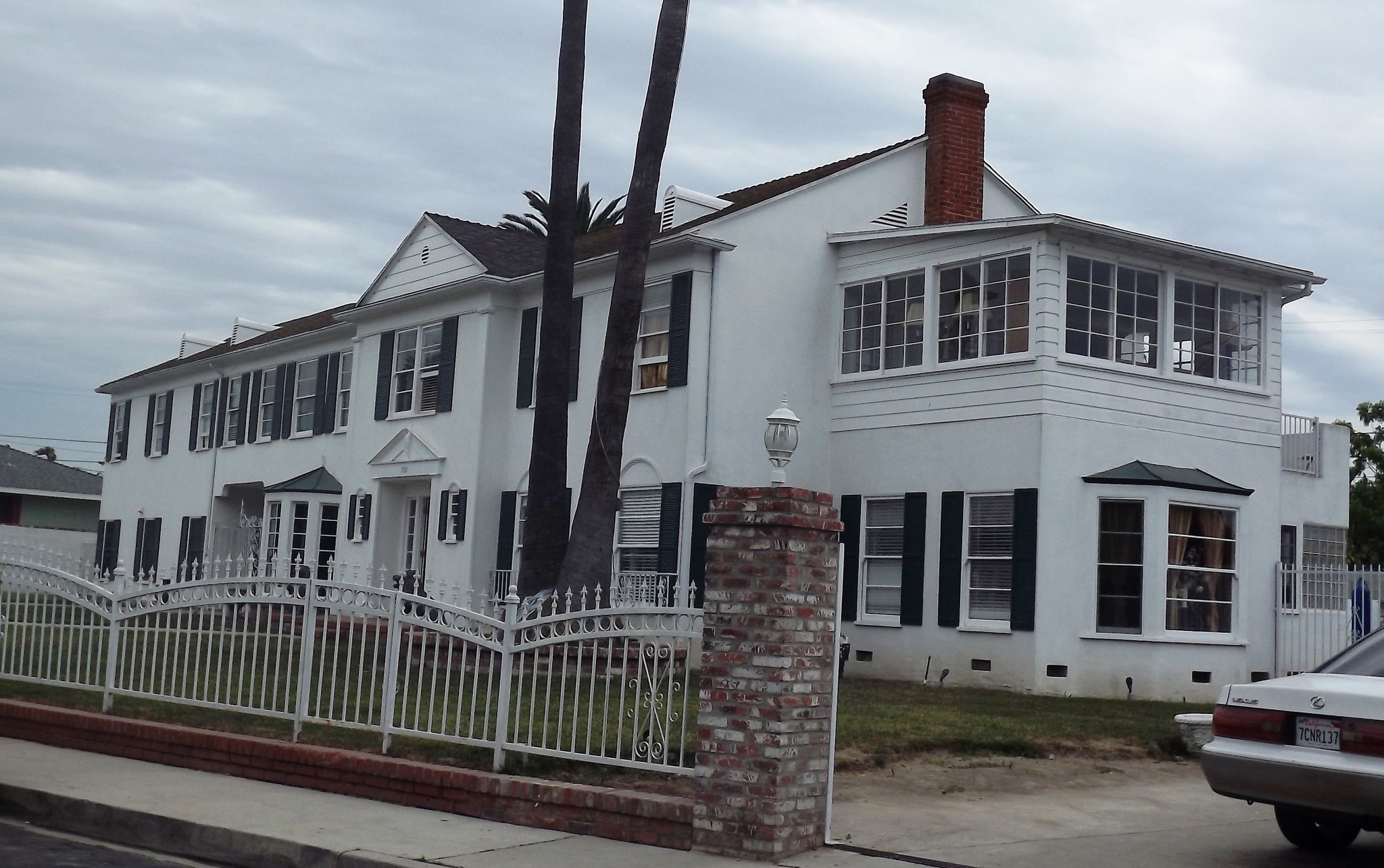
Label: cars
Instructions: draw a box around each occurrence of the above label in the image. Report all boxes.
[1200,623,1384,854]
